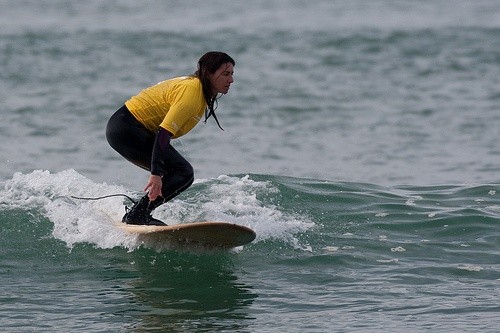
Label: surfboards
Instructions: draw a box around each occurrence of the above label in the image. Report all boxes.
[113,195,256,249]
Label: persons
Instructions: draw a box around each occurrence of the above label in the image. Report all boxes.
[106,51,236,226]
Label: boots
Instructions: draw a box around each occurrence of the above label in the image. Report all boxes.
[124,192,167,226]
